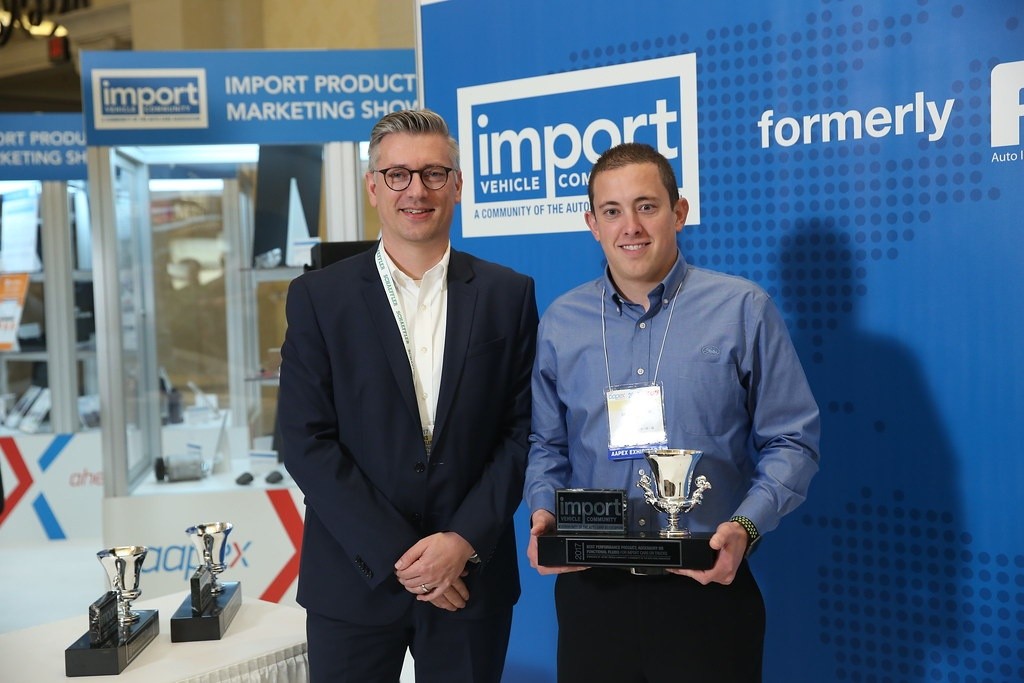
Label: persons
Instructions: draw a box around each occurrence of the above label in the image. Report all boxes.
[278,109,540,683]
[523,143,820,683]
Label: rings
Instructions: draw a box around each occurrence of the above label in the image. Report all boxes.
[422,585,429,593]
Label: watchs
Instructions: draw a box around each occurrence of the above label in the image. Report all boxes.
[730,516,761,558]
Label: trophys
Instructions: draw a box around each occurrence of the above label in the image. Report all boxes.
[170,523,242,642]
[537,449,719,570]
[65,546,160,677]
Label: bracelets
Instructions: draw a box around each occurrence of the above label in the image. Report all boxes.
[470,553,478,559]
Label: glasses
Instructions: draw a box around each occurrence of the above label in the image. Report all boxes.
[374,166,458,192]
